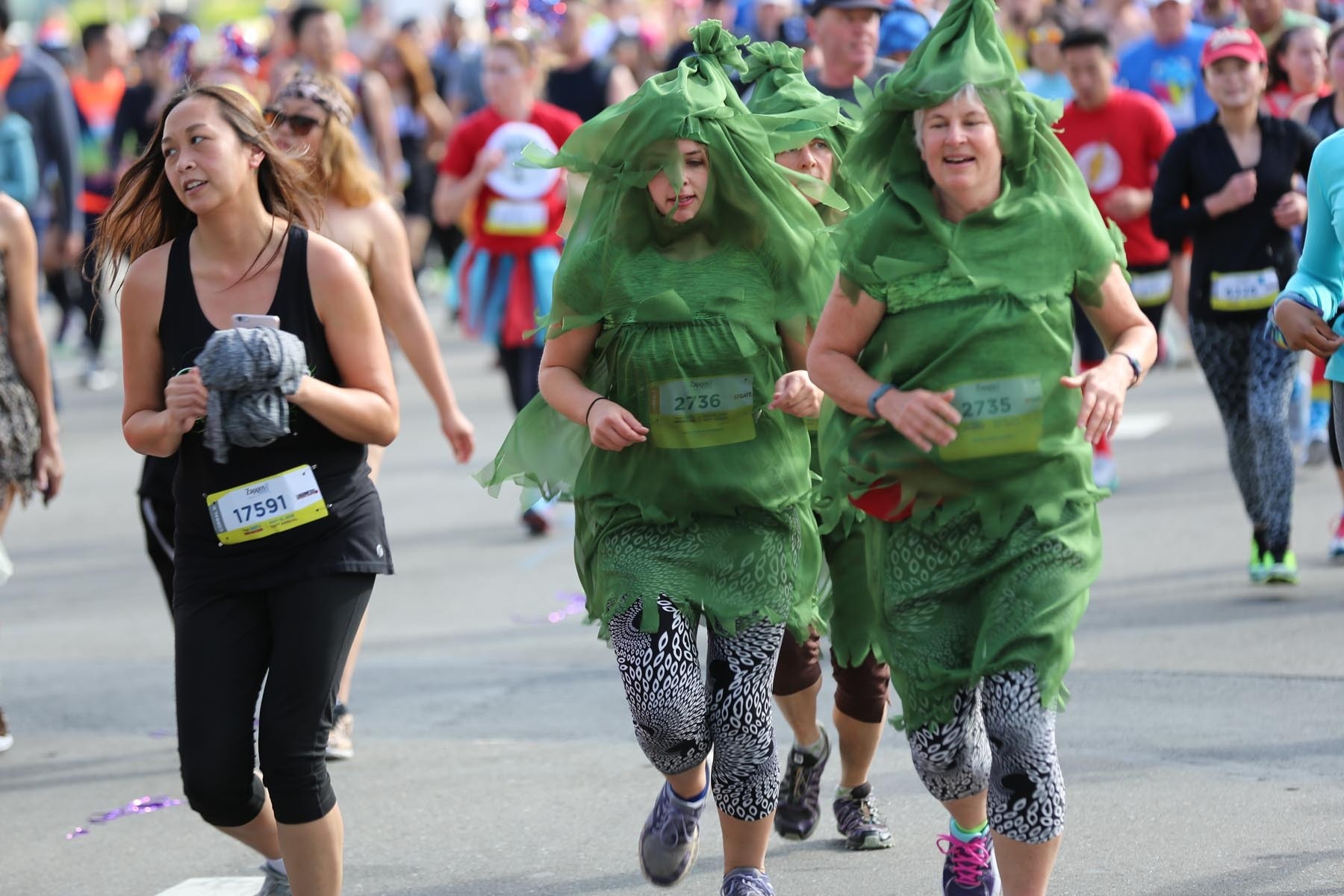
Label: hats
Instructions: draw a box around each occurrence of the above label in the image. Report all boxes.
[1200,27,1268,67]
[802,0,891,14]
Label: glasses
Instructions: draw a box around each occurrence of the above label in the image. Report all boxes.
[262,108,327,138]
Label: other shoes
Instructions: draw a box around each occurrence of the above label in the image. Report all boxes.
[324,719,352,760]
[524,498,551,537]
[255,864,292,895]
[1089,455,1111,493]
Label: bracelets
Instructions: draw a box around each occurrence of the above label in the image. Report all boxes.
[586,396,609,424]
[1111,351,1142,389]
[867,382,895,419]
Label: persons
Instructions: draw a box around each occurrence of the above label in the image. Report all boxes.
[471,18,842,896]
[805,0,1159,896]
[0,0,1344,584]
[83,85,400,896]
[1268,127,1344,472]
[740,39,902,852]
[0,192,63,752]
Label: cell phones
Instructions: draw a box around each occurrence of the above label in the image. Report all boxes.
[231,315,280,330]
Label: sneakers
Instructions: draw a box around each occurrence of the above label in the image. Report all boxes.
[1251,533,1273,582]
[935,825,1001,895]
[1328,511,1344,553]
[639,760,710,887]
[775,721,832,841]
[834,782,894,850]
[1267,551,1296,582]
[720,868,771,896]
